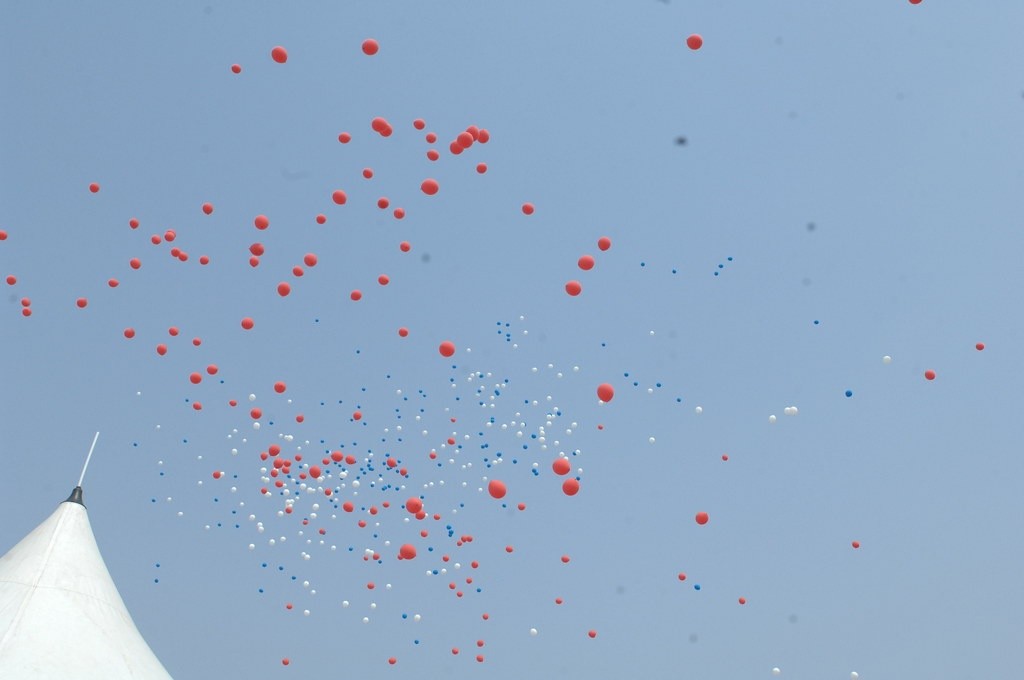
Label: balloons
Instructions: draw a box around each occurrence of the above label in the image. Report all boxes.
[361,38,379,55]
[247,118,491,302]
[231,64,241,73]
[908,0,923,4]
[638,256,733,279]
[564,236,611,295]
[685,33,703,50]
[271,46,288,63]
[522,203,535,215]
[0,229,31,316]
[119,315,986,679]
[77,182,213,307]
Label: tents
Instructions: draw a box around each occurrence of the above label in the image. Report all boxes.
[0,430,174,680]
